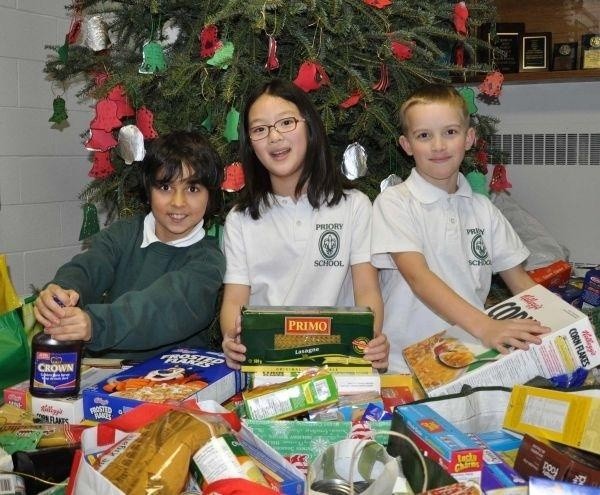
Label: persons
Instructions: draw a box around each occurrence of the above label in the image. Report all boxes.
[31,131,222,358]
[221,76,390,370]
[373,86,550,372]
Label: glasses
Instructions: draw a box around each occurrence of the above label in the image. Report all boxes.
[246,115,311,143]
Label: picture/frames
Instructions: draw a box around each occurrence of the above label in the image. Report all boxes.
[480,20,526,75]
[517,30,553,73]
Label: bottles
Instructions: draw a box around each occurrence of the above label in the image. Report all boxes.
[29,298,83,399]
